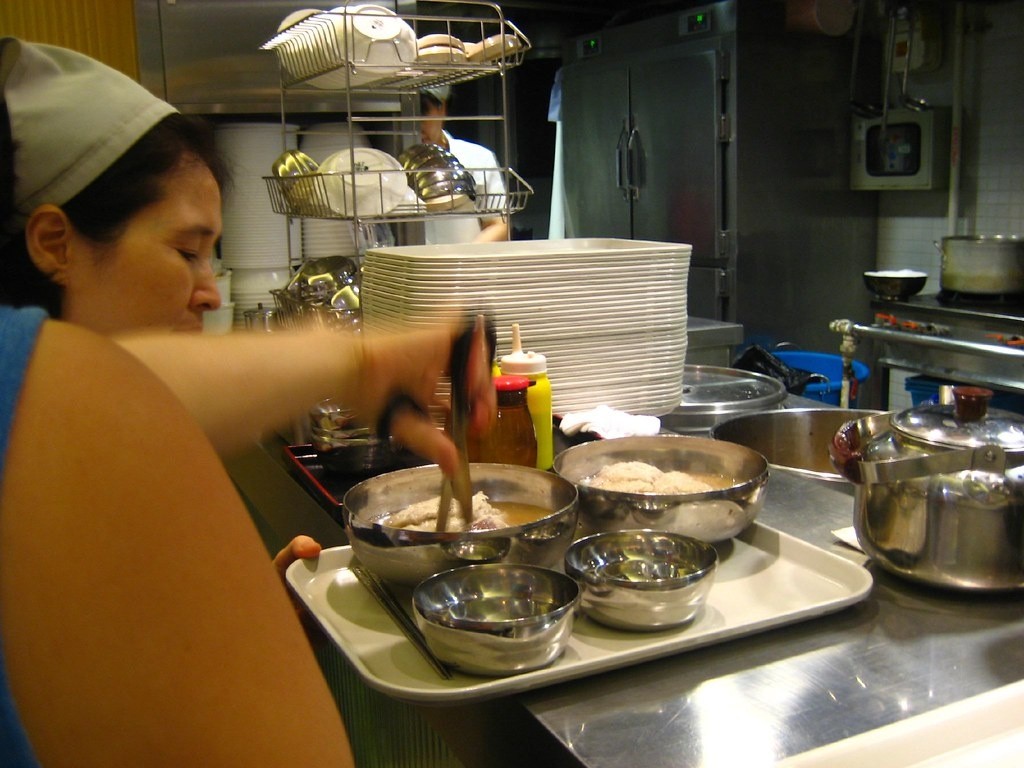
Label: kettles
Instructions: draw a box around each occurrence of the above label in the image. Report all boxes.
[829,386,1024,592]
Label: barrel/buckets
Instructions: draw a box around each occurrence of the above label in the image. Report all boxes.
[735,341,870,411]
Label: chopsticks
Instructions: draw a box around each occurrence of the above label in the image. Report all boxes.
[352,566,453,678]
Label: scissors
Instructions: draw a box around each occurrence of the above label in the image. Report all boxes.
[380,318,498,530]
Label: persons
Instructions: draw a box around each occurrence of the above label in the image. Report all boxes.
[420,82,509,245]
[0,32,499,768]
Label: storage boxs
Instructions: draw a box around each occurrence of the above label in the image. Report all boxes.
[885,369,1024,413]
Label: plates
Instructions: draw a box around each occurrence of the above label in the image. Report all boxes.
[361,237,692,441]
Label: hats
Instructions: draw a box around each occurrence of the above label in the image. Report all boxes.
[420,84,451,104]
[0,37,182,239]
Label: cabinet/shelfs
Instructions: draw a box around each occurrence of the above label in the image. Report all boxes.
[252,0,533,328]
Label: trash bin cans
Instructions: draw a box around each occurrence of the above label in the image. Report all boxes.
[729,344,870,409]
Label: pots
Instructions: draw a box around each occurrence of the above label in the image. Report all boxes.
[933,235,1024,300]
[708,409,890,480]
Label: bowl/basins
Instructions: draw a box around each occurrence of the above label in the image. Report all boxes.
[343,462,580,590]
[310,397,402,475]
[202,123,476,340]
[275,4,417,90]
[413,563,580,677]
[863,271,927,300]
[566,529,718,632]
[551,436,769,544]
